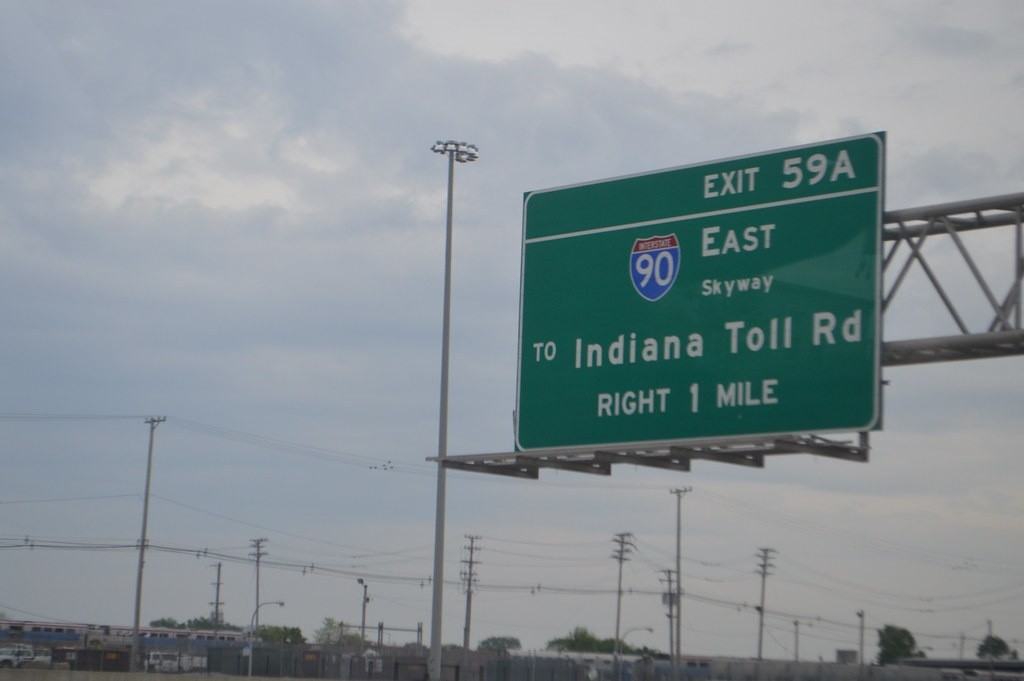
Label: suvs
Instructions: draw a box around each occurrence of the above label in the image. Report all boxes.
[0,649,34,669]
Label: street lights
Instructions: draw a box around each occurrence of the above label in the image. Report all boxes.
[248,601,285,680]
[355,576,369,656]
[422,141,483,680]
[618,626,656,681]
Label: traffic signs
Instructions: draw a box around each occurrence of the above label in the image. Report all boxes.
[513,130,889,462]
